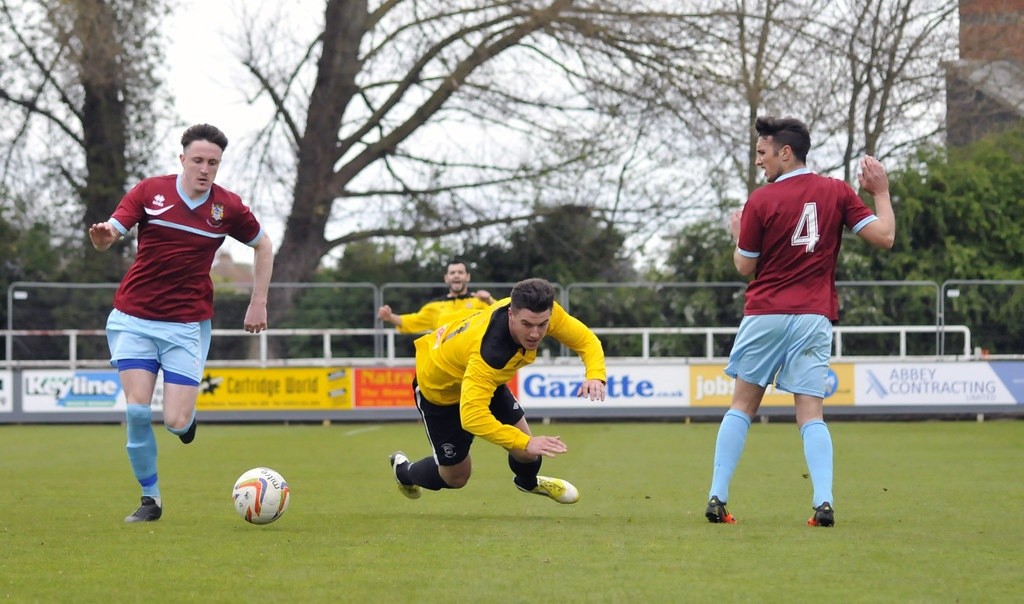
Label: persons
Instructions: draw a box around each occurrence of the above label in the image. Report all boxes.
[389,281,608,505]
[705,119,897,529]
[87,122,274,521]
[378,260,498,337]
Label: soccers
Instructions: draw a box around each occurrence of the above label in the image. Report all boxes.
[232,466,291,525]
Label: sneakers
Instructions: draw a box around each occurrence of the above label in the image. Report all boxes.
[808,502,835,527]
[180,412,199,443]
[514,476,581,505]
[389,452,422,498]
[705,496,737,523]
[124,495,161,524]
